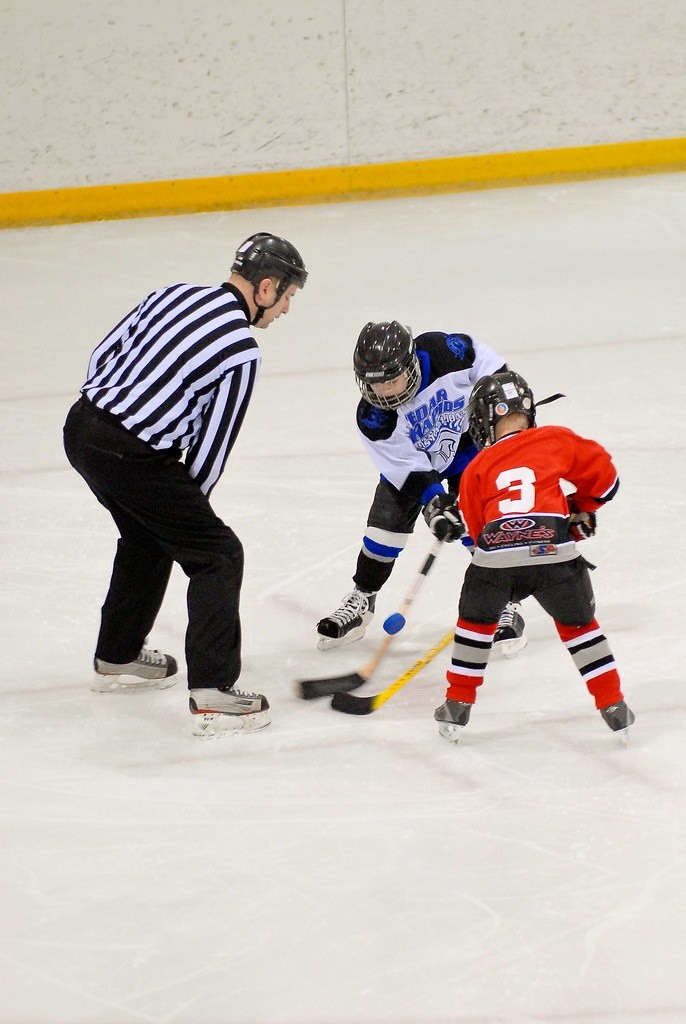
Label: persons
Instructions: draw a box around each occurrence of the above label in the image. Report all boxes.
[63,233,308,742]
[316,320,528,656]
[435,371,635,747]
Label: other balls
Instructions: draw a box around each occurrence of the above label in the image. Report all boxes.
[382,612,406,635]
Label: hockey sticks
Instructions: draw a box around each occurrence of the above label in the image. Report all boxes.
[327,511,590,715]
[292,490,461,703]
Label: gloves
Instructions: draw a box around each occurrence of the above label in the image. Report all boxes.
[567,512,597,537]
[422,492,466,543]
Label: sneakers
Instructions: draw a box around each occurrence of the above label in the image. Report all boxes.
[188,685,273,739]
[434,698,473,746]
[315,585,377,651]
[493,600,529,656]
[600,699,637,748]
[89,637,182,695]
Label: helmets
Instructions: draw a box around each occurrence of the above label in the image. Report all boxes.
[352,319,417,408]
[468,370,536,452]
[230,232,310,296]
